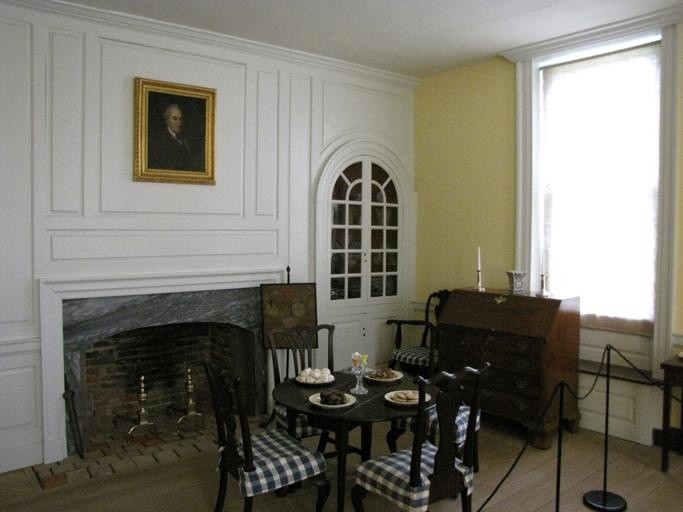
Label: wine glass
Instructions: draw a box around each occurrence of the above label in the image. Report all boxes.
[350,353,371,396]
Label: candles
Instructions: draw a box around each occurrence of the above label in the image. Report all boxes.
[478,246,481,271]
[540,249,544,275]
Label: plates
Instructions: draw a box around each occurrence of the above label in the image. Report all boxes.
[295,375,336,385]
[365,368,405,382]
[384,389,432,405]
[308,392,357,409]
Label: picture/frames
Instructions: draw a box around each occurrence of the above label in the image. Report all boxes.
[134,77,217,185]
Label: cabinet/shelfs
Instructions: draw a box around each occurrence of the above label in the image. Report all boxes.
[437,286,582,450]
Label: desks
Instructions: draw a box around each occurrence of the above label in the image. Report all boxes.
[658,357,682,472]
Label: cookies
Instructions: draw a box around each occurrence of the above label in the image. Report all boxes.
[390,391,417,403]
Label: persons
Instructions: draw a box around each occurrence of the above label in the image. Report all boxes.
[149,102,204,172]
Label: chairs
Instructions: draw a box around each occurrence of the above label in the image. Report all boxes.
[348,360,491,512]
[383,289,438,388]
[201,359,330,511]
[384,327,490,474]
[267,321,359,460]
